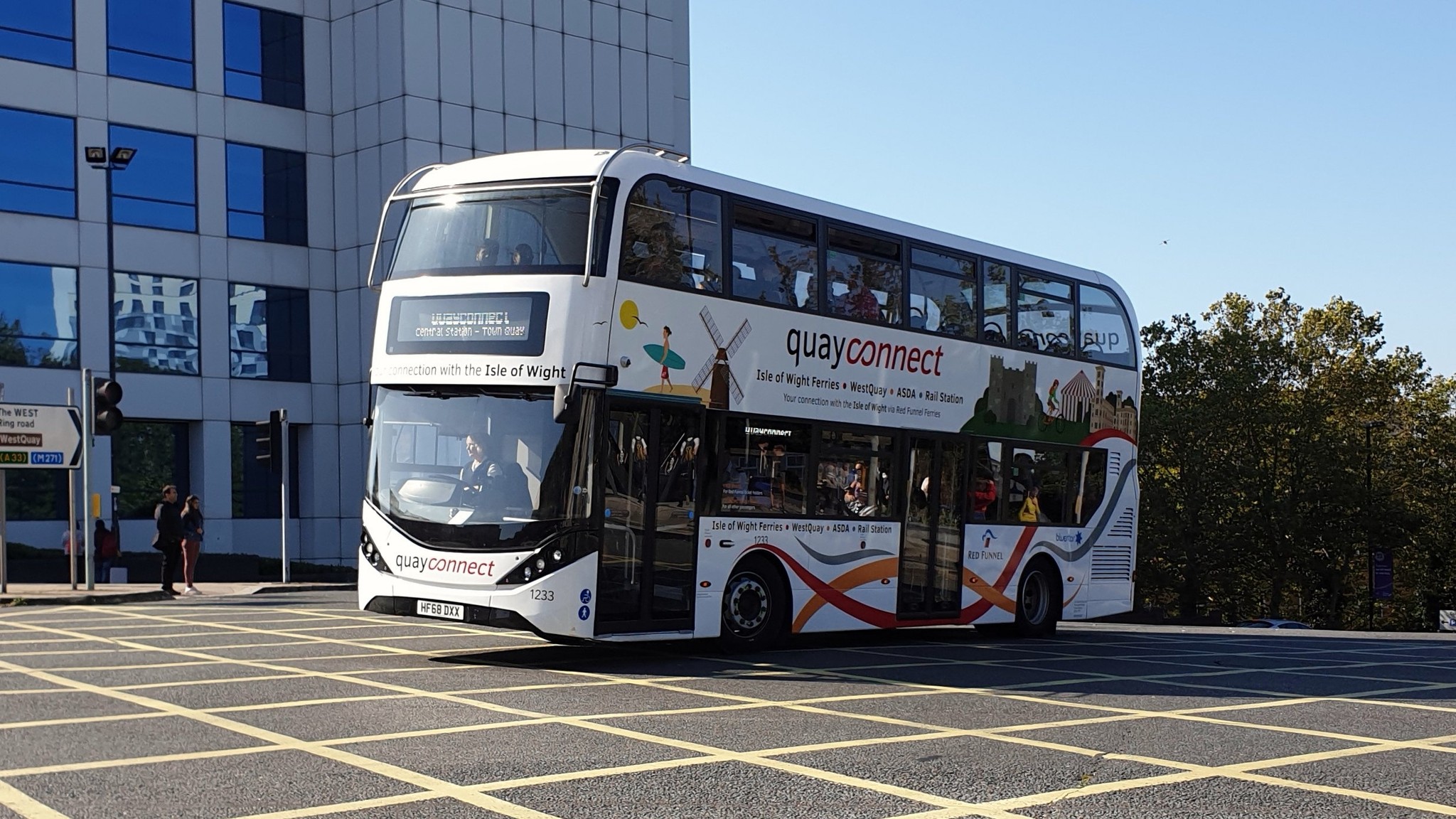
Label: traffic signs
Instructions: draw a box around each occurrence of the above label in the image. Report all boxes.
[1439,609,1456,631]
[0,402,86,470]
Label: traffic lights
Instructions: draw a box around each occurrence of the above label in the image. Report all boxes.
[255,410,282,478]
[92,376,123,436]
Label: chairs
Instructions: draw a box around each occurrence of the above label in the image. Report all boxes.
[877,303,1104,361]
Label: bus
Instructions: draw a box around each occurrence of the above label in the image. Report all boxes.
[357,144,1141,654]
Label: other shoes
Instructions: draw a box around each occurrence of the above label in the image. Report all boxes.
[185,587,202,595]
[163,587,181,595]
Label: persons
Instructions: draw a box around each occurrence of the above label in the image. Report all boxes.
[1018,487,1040,522]
[94,520,121,583]
[151,485,184,599]
[723,446,749,506]
[767,443,889,517]
[622,222,1056,351]
[967,476,996,521]
[511,238,547,265]
[181,494,205,595]
[453,432,503,509]
[475,238,500,266]
[62,520,85,583]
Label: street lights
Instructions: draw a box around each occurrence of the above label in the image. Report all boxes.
[84,145,137,565]
[1365,421,1387,631]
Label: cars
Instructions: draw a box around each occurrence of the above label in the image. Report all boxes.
[1237,620,1316,630]
